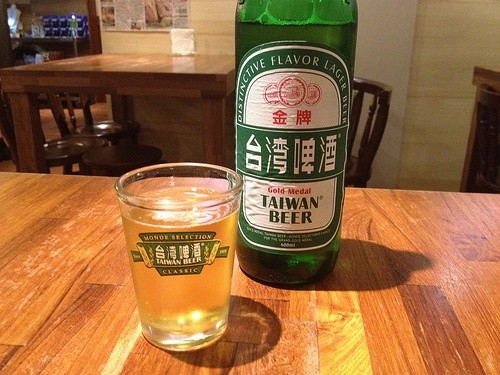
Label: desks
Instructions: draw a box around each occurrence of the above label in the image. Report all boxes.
[0,170,500,375]
[3,37,90,104]
[0,53,236,172]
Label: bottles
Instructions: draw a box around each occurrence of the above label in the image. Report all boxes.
[234,0,358,286]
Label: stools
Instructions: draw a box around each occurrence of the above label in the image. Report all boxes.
[83,144,163,177]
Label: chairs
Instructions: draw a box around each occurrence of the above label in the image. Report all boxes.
[458,66,500,195]
[343,78,393,188]
[0,91,95,174]
[46,91,139,145]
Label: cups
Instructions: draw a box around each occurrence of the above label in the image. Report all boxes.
[114,162,243,352]
